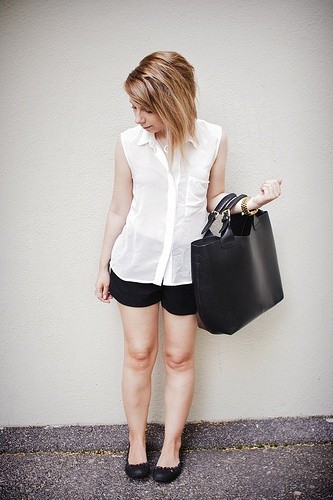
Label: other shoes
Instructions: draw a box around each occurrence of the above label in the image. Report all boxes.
[152,446,184,483]
[126,442,150,479]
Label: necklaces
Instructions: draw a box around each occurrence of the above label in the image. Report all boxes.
[157,133,169,154]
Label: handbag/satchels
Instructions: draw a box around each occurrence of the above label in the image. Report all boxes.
[191,193,284,335]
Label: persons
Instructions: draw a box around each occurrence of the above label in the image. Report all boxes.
[94,52,282,484]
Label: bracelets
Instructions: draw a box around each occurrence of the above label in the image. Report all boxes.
[239,196,258,216]
